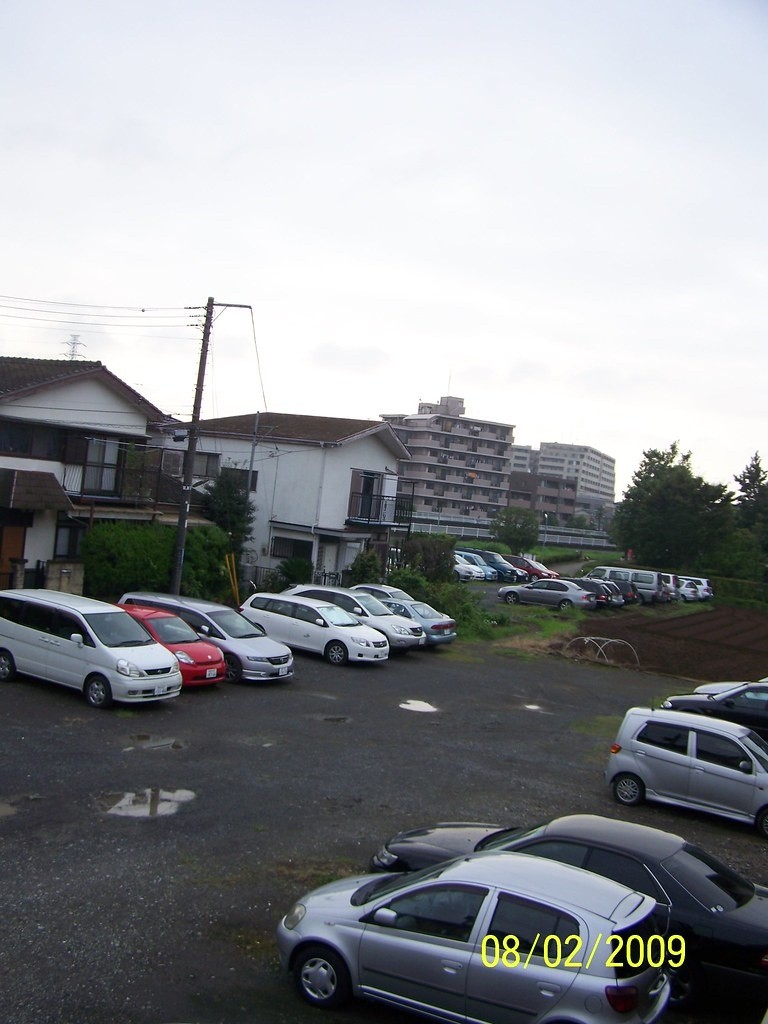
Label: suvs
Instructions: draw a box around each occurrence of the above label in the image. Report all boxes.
[460,549,516,582]
[281,584,426,656]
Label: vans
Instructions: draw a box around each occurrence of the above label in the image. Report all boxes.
[606,706,768,836]
[659,572,680,603]
[117,591,295,684]
[582,566,662,605]
[678,575,713,601]
[0,587,182,708]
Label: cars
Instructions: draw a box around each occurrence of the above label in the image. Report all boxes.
[369,815,768,1015]
[349,583,414,604]
[512,565,528,581]
[677,579,699,602]
[378,598,457,648]
[114,603,227,688]
[497,578,638,612]
[447,550,485,582]
[661,677,768,742]
[500,553,560,583]
[238,593,390,665]
[279,850,671,1024]
